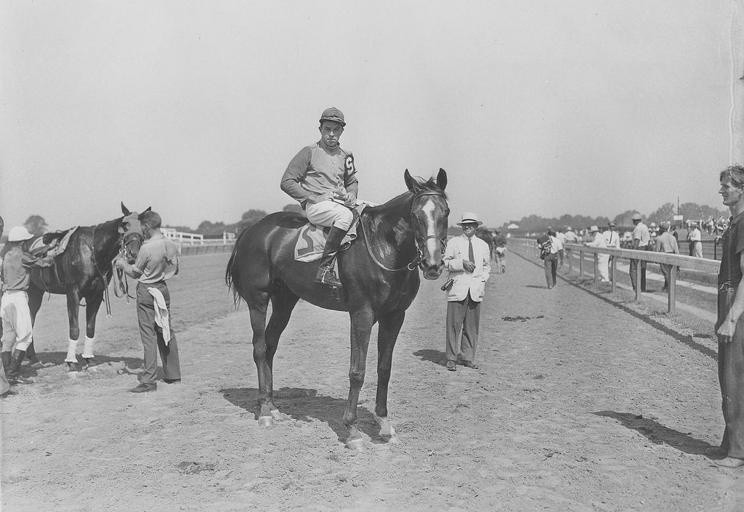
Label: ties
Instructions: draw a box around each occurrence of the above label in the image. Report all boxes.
[468,239,475,265]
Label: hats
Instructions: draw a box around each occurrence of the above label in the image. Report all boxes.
[457,213,483,226]
[608,222,617,226]
[632,214,642,220]
[589,226,600,233]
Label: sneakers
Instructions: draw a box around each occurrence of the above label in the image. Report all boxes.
[705,446,728,458]
[715,458,744,467]
[447,361,456,371]
[461,360,479,369]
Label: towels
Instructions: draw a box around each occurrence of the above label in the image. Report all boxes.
[148,288,171,346]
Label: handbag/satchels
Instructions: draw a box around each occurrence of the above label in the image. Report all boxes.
[537,235,551,259]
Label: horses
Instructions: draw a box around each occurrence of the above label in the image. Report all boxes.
[225,166,450,451]
[0,201,152,379]
[475,225,494,262]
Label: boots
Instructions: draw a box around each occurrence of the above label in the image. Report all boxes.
[314,226,346,289]
[1,352,11,376]
[8,349,32,385]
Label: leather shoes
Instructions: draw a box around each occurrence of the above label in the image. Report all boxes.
[161,376,181,384]
[131,383,157,393]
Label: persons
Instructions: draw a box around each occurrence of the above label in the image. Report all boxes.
[280,106,359,290]
[1,217,56,398]
[443,212,492,373]
[538,227,564,290]
[705,163,744,469]
[657,222,680,292]
[492,227,508,275]
[546,215,732,281]
[115,211,182,393]
[629,213,650,295]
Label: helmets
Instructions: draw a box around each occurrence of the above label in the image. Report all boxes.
[319,107,347,126]
[8,227,35,241]
